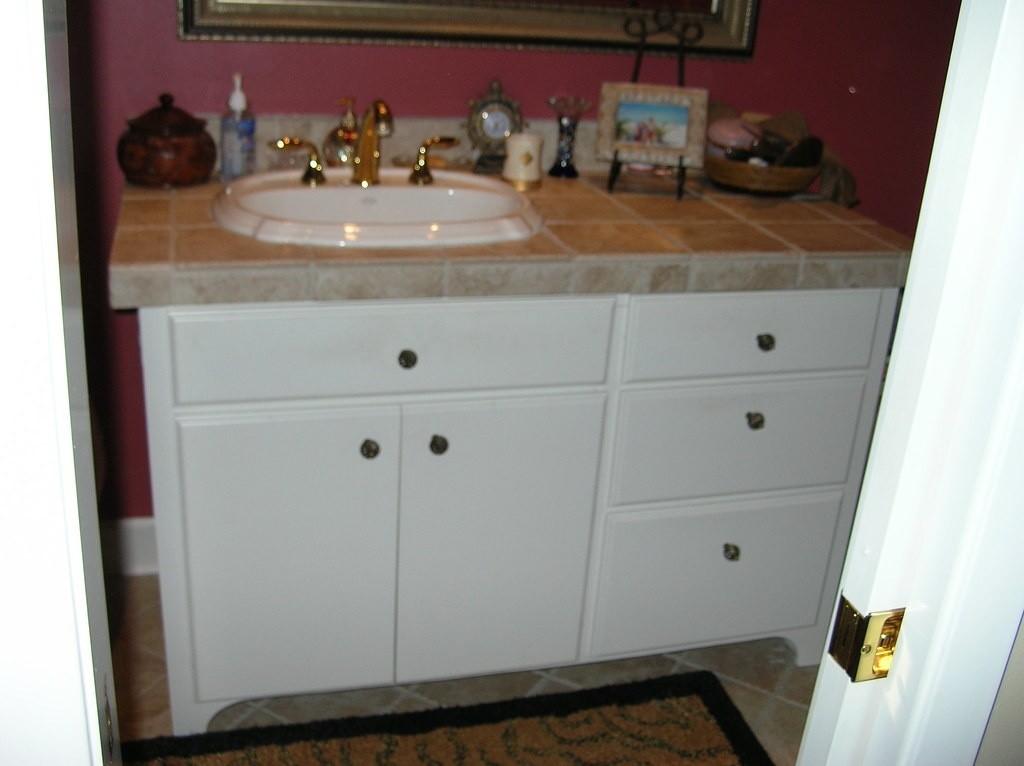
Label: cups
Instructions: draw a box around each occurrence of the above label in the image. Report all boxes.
[501,133,543,190]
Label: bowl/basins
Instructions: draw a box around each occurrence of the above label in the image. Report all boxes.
[704,155,825,194]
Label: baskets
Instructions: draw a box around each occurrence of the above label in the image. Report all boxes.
[703,150,823,194]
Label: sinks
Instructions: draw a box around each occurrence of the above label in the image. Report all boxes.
[209,164,543,249]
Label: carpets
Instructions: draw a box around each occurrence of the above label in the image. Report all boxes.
[127,670,776,765]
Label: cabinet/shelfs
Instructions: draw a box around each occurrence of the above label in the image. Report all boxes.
[139,287,899,736]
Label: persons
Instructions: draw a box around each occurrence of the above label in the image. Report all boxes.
[634,115,657,145]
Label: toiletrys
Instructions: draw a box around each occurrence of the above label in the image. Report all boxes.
[321,95,359,166]
[217,70,255,186]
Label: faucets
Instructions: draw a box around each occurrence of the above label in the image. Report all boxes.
[349,98,395,187]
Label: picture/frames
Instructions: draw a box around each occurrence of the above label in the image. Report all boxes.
[593,82,710,171]
